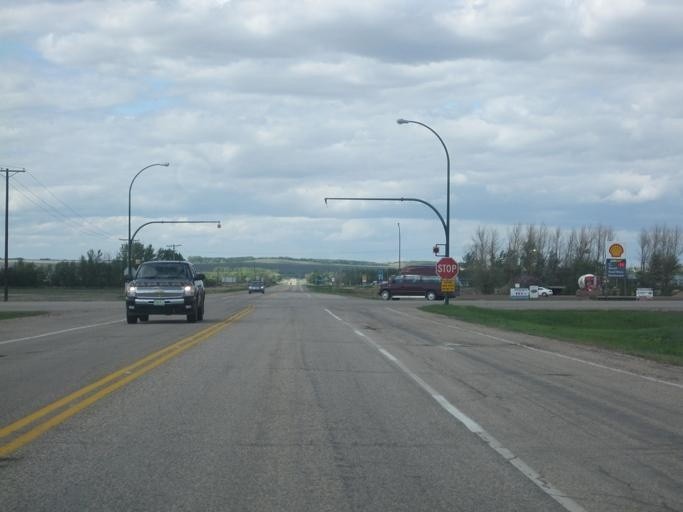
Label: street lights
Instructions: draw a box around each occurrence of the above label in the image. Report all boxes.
[394,119,451,305]
[395,221,401,272]
[126,161,170,278]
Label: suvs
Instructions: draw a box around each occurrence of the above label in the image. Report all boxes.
[248,280,265,294]
[125,260,205,323]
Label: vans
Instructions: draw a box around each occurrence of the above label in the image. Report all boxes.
[377,265,460,301]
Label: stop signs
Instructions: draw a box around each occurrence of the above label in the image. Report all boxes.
[435,257,458,279]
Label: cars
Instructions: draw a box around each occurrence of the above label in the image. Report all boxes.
[538,286,554,297]
